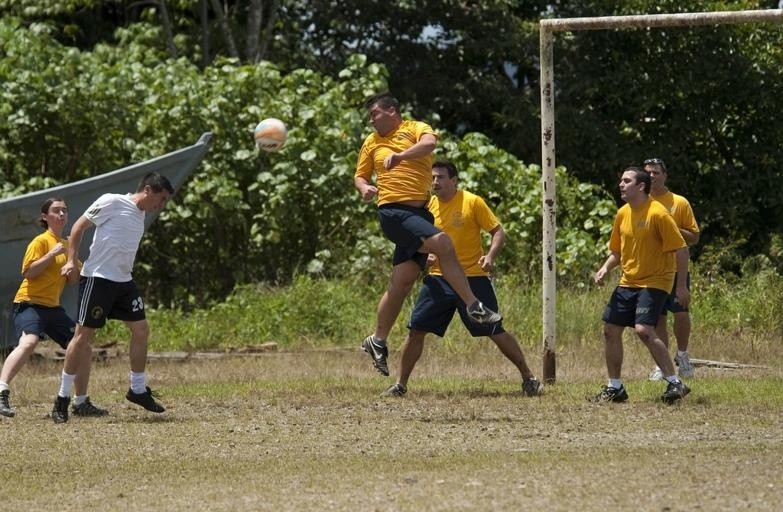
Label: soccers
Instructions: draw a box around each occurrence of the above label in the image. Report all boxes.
[254,118,287,151]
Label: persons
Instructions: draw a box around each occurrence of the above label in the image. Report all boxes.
[385,162,541,397]
[643,158,700,381]
[354,94,503,376]
[52,170,174,423]
[594,167,692,400]
[0,199,110,415]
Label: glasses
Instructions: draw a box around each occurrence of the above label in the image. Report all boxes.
[643,158,665,170]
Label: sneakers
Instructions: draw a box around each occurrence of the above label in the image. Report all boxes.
[674,351,694,380]
[464,299,503,324]
[125,386,166,414]
[361,334,390,377]
[522,378,544,396]
[647,368,662,381]
[52,393,70,424]
[660,379,692,403]
[71,396,110,418]
[0,389,16,418]
[385,382,407,397]
[593,384,628,403]
